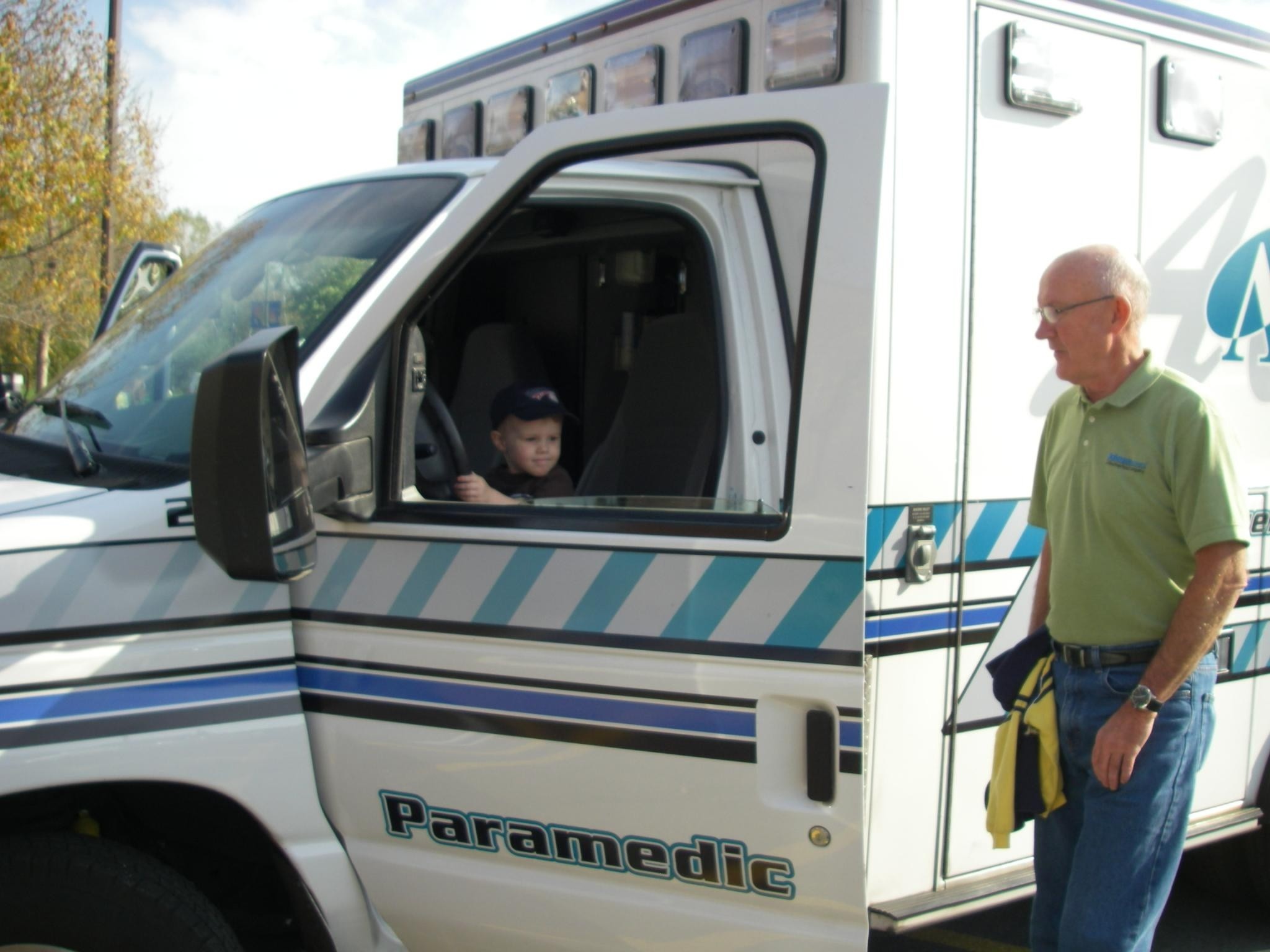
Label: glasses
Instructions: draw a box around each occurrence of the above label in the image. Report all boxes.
[1034,295,1118,324]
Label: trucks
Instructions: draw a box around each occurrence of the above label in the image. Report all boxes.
[1,4,1267,948]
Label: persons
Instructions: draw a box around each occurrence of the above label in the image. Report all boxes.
[454,388,576,504]
[980,244,1251,950]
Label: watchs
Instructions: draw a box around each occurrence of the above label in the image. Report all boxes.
[1130,683,1163,712]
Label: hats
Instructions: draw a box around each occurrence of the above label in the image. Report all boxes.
[490,381,580,428]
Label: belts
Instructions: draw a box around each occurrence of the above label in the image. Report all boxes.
[1051,637,1157,668]
